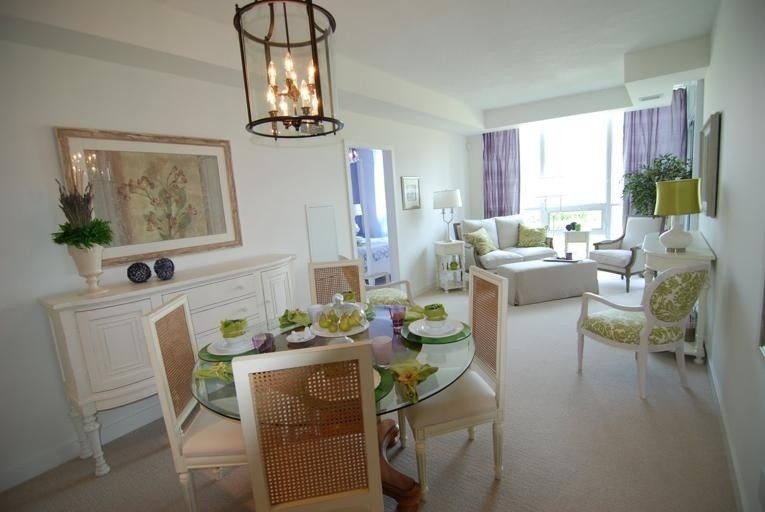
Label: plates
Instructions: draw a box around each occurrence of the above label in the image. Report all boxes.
[286,330,316,344]
[401,323,471,344]
[206,328,266,355]
[407,318,464,338]
[297,369,395,411]
[197,344,254,363]
[306,364,381,400]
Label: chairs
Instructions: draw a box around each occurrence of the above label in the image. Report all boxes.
[576,263,705,400]
[338,254,415,306]
[589,216,665,293]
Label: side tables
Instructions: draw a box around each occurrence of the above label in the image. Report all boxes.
[565,231,589,259]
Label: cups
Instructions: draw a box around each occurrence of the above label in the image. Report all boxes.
[252,333,272,352]
[388,306,405,328]
[372,336,392,367]
[308,304,322,323]
[566,253,572,260]
[575,224,581,231]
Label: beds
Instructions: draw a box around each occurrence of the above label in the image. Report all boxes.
[357,237,391,285]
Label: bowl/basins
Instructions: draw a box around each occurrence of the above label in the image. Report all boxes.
[222,331,248,345]
[424,315,447,329]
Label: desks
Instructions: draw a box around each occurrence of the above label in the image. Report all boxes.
[38,253,296,477]
[642,231,716,364]
[435,240,467,293]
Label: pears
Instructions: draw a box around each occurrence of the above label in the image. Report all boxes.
[320,308,361,333]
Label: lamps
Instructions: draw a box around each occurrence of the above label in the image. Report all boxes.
[654,179,702,253]
[353,204,363,236]
[433,190,462,242]
[234,0,344,140]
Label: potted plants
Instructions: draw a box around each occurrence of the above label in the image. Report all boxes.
[50,178,114,297]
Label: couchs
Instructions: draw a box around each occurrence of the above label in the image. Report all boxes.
[454,214,557,282]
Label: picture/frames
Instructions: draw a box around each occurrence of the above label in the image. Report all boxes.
[698,113,720,218]
[401,177,421,210]
[53,125,243,268]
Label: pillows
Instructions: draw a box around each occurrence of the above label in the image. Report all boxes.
[517,223,548,247]
[464,227,496,255]
